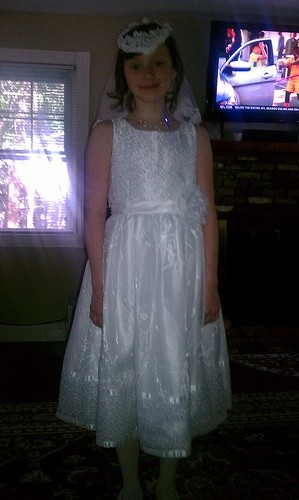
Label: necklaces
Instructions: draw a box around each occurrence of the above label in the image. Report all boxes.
[126,110,167,131]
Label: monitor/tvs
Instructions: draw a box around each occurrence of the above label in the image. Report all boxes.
[206,21,299,124]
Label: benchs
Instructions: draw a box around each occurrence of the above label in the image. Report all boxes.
[0,246,89,342]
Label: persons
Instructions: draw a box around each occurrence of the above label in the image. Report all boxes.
[224,27,299,108]
[53,18,235,500]
